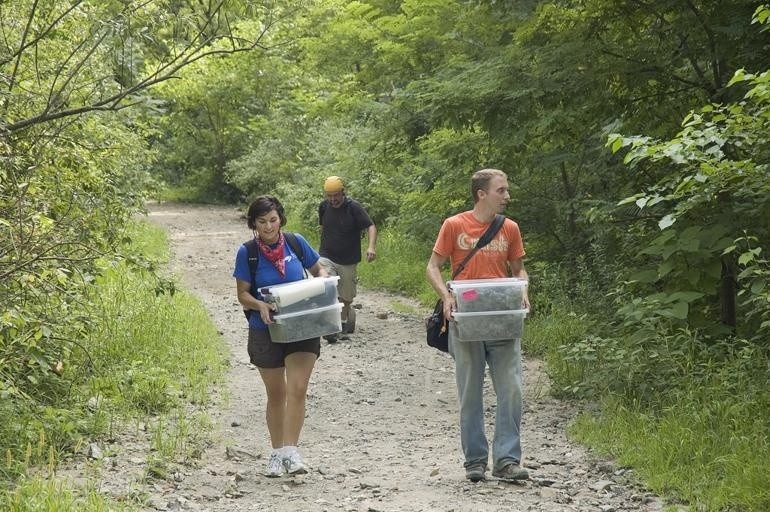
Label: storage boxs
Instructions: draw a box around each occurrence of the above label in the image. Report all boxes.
[446,277,529,342]
[258,274,345,343]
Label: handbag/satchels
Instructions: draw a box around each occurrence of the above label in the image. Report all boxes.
[427,298,449,352]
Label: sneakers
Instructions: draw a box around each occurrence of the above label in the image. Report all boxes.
[327,320,348,342]
[466,465,486,482]
[264,450,282,477]
[280,452,309,475]
[492,463,529,480]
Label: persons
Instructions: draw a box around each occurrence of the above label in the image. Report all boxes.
[316,175,377,345]
[232,194,331,478]
[423,169,532,481]
